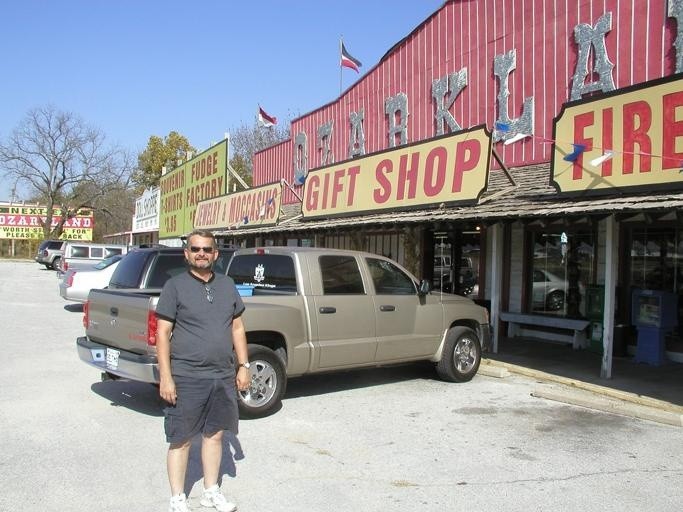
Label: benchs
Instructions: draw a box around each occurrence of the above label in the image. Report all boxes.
[501,311,592,350]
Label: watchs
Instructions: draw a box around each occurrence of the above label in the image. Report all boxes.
[239,362,250,369]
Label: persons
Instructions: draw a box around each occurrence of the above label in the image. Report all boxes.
[155,230,251,512]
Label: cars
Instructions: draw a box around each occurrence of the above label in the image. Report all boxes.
[463,269,585,310]
[34,240,236,302]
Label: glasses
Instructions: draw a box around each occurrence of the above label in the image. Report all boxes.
[189,245,214,253]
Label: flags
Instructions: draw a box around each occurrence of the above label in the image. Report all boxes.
[259,108,277,128]
[564,145,585,161]
[267,198,272,205]
[340,41,362,73]
[503,133,531,146]
[259,204,265,216]
[236,223,240,228]
[228,225,232,230]
[244,216,248,224]
[296,175,304,184]
[590,149,615,168]
[495,123,508,138]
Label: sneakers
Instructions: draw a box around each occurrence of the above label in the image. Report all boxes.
[169,493,192,512]
[199,483,237,512]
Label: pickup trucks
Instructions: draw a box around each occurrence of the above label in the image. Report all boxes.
[434,254,473,280]
[76,246,491,418]
